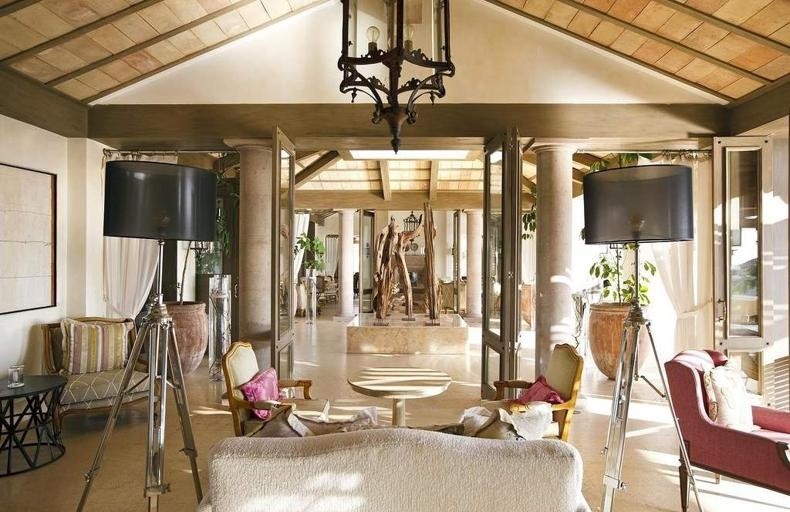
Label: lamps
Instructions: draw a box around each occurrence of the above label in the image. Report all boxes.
[76,157,217,511]
[402,210,421,237]
[581,163,703,511]
[337,0,455,152]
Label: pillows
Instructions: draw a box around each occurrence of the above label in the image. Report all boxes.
[702,353,760,433]
[58,317,135,376]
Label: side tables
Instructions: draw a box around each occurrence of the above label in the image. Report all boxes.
[0,375,68,478]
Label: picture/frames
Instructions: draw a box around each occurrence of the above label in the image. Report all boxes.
[0,162,56,316]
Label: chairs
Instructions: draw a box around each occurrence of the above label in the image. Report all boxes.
[662,347,790,511]
[40,316,161,443]
[222,341,312,436]
[480,343,584,442]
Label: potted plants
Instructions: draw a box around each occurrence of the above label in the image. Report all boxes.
[588,151,656,381]
[143,156,239,378]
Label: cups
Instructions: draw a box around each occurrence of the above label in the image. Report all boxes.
[8,364,24,388]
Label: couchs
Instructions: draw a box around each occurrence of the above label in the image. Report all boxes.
[195,427,594,511]
